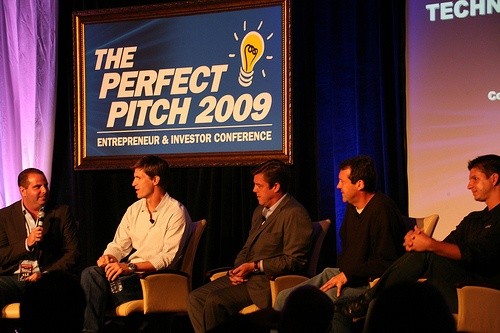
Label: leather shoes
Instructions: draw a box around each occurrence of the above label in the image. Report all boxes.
[344,294,369,320]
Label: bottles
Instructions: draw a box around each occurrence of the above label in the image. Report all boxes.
[104,260,124,294]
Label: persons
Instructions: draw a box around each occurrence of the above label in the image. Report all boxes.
[80,154,193,333]
[347,154,500,333]
[187,159,314,333]
[274,155,404,333]
[0,168,80,333]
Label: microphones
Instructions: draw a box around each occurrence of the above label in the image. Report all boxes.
[35,211,45,247]
[261,215,265,222]
[151,220,154,224]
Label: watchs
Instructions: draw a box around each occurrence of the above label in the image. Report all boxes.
[252,261,259,273]
[127,263,136,273]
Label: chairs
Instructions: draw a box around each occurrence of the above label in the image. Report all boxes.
[0,214,500,333]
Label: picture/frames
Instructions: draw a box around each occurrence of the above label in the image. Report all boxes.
[70,0,294,168]
